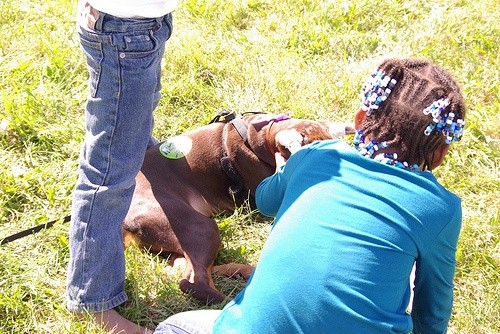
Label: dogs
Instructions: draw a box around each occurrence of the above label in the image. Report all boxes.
[122,114,356,306]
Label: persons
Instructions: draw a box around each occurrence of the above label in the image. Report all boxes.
[68,1,176,334]
[154,58,466,334]
